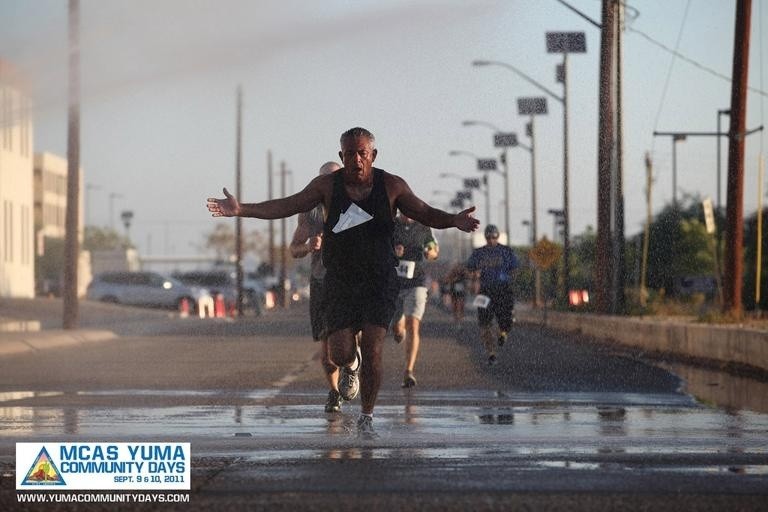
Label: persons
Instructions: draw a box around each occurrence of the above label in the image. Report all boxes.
[207,127,477,439]
[467,224,519,368]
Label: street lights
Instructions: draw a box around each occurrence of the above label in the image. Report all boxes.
[717,108,730,249]
[673,132,685,204]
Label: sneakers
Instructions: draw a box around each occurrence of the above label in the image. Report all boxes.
[498,331,507,347]
[357,416,380,440]
[337,347,361,400]
[488,353,497,365]
[401,375,416,388]
[325,391,342,412]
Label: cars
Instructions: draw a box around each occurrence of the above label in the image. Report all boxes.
[87,261,292,317]
